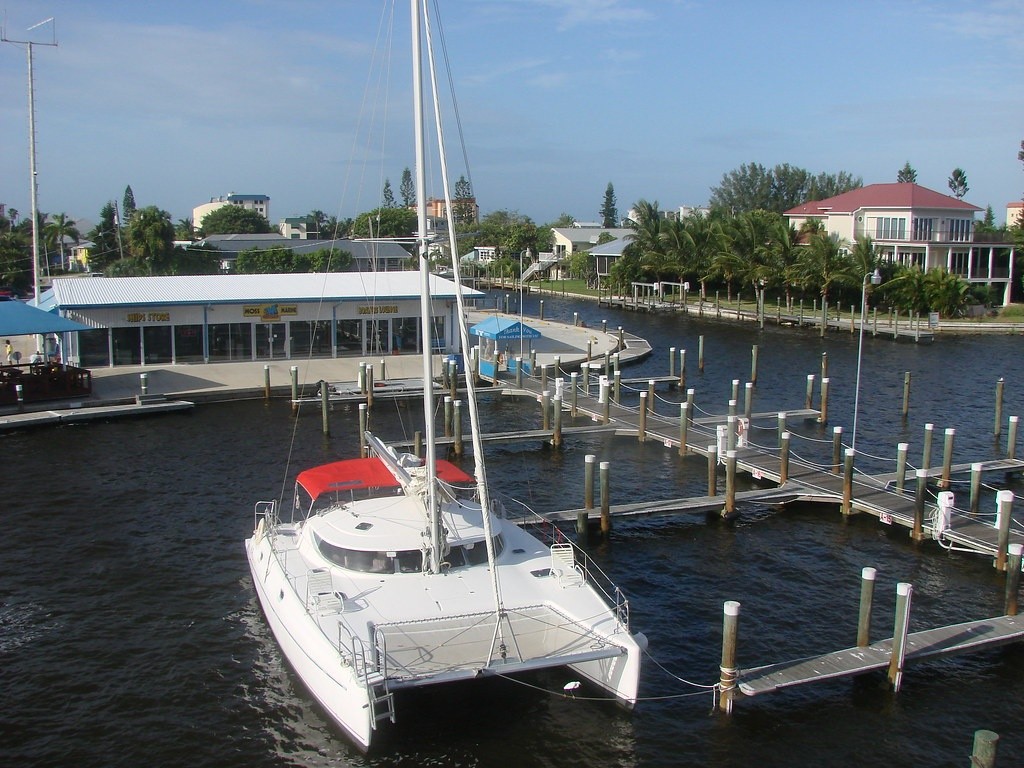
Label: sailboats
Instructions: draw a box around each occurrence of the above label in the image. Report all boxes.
[244,2,645,757]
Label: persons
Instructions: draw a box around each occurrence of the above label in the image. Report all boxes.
[5,340,13,364]
[29,351,42,367]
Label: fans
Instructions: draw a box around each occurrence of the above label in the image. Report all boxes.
[12,351,22,370]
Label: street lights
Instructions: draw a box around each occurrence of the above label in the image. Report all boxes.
[519,249,532,361]
[852,269,881,450]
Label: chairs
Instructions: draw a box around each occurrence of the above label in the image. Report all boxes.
[549,543,585,589]
[305,566,344,617]
